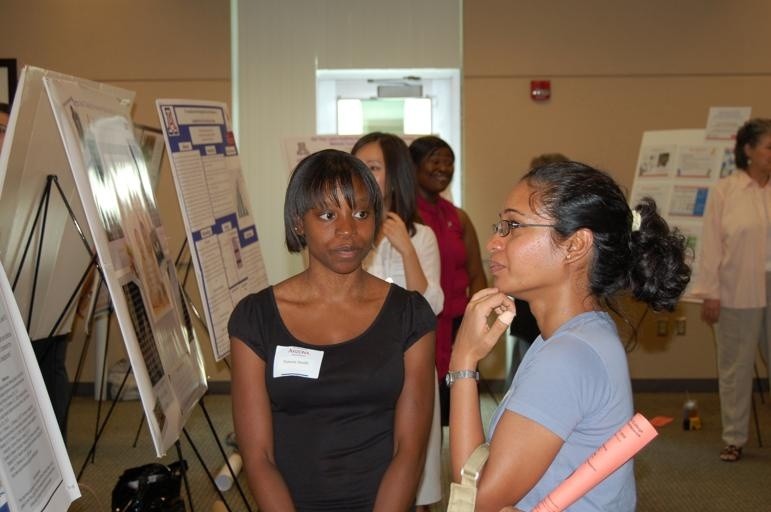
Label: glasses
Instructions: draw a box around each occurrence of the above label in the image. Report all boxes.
[492,221,552,236]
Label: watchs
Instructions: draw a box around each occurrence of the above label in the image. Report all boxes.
[445,369,479,388]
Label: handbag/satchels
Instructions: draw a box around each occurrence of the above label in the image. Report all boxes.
[110,460,188,512]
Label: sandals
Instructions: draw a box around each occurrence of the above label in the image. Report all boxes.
[720,445,743,461]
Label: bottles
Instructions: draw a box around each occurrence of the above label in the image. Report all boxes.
[684,402,703,430]
[215,452,243,492]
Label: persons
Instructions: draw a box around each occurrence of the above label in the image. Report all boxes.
[227,149,436,511]
[700,117,771,462]
[408,137,489,425]
[445,151,694,512]
[350,133,444,511]
